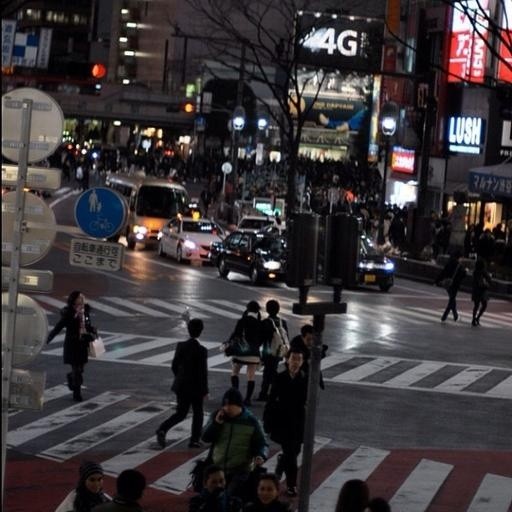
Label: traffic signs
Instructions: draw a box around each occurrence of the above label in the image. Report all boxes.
[196,117,206,131]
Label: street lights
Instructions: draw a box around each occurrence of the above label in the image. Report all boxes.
[375,97,401,244]
[228,105,248,204]
[257,109,271,137]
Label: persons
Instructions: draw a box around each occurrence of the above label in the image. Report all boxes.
[290,324,329,446]
[92,467,149,512]
[430,250,466,324]
[334,479,370,511]
[201,387,271,498]
[252,299,292,403]
[186,457,244,512]
[362,495,391,511]
[26,140,120,200]
[125,139,511,264]
[72,460,113,511]
[154,317,211,450]
[262,349,310,497]
[220,300,263,407]
[242,474,294,511]
[469,259,495,327]
[46,291,99,402]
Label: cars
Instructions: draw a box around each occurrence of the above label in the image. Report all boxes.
[159,211,399,296]
[65,136,188,174]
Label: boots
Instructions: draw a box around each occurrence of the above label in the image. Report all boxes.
[244,381,255,406]
[231,375,239,389]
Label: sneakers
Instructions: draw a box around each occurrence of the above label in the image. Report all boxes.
[189,442,203,448]
[286,486,298,494]
[441,320,447,322]
[157,430,166,446]
[473,321,477,325]
[476,317,479,325]
[455,315,460,322]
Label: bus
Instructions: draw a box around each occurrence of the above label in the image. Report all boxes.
[105,173,194,251]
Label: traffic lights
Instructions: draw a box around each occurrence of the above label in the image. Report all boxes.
[86,60,107,83]
[182,100,198,115]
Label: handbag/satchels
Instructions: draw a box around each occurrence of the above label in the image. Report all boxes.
[268,329,291,357]
[88,335,105,358]
[442,279,453,287]
[228,336,248,355]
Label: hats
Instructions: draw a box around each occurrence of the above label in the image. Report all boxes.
[79,460,103,479]
